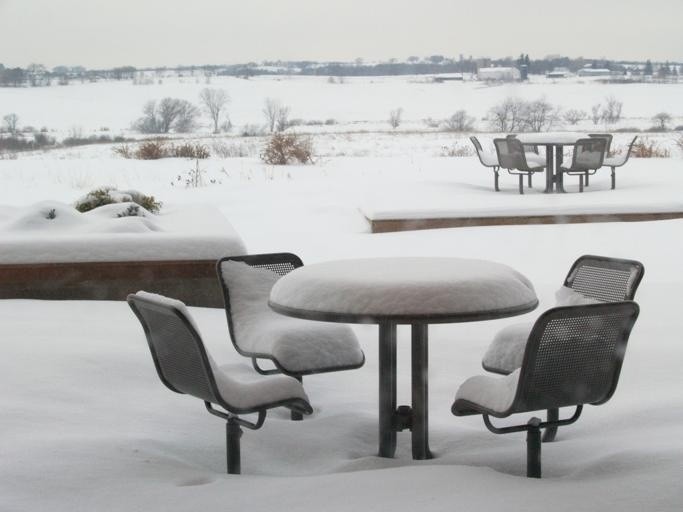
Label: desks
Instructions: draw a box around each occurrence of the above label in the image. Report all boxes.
[520,140,577,195]
[268,294,539,460]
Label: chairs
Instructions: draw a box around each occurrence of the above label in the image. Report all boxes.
[126,294,313,475]
[482,255,645,442]
[506,135,546,188]
[585,133,613,186]
[560,138,607,192]
[494,138,544,194]
[469,135,509,192]
[602,136,638,189]
[451,299,640,479]
[217,253,365,421]
